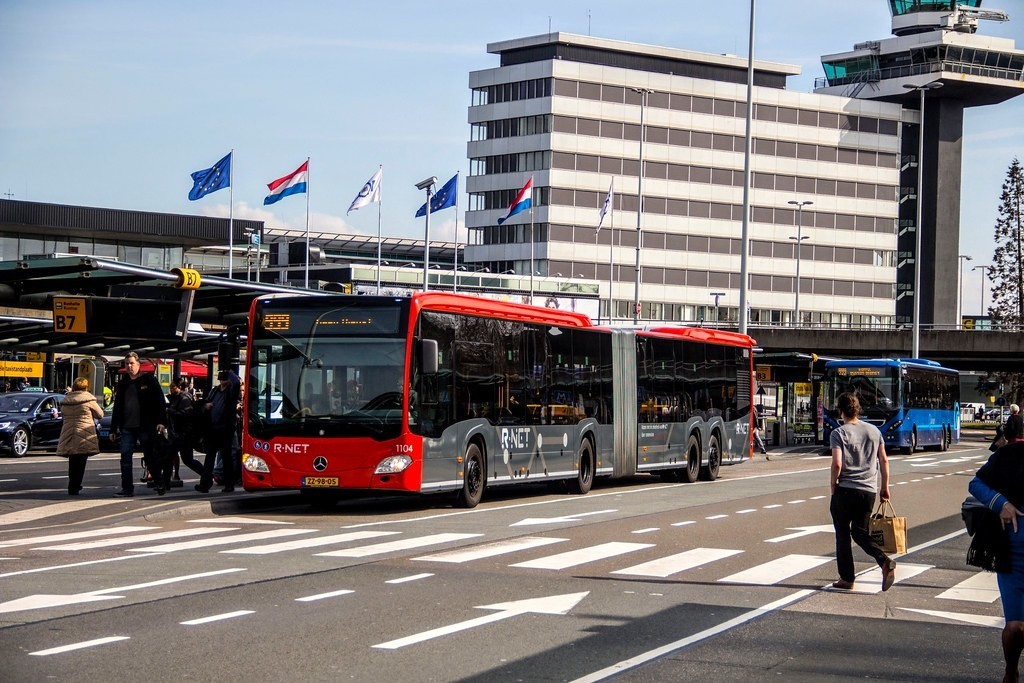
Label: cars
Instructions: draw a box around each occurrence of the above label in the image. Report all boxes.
[0,387,67,457]
[975,407,1011,422]
[96,398,123,452]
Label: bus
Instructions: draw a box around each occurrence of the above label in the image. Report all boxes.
[822,357,962,455]
[240,289,757,509]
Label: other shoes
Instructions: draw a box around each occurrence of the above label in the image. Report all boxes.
[221,486,235,493]
[195,483,209,493]
[156,478,166,495]
[833,577,854,589]
[113,488,134,497]
[882,559,896,591]
[211,473,215,488]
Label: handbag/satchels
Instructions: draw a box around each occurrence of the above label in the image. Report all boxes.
[869,499,907,555]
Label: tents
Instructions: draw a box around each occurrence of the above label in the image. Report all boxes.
[118,358,207,381]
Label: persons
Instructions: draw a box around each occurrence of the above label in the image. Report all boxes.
[303,379,359,412]
[396,376,418,420]
[1005,403,1024,440]
[979,405,983,421]
[56,377,105,496]
[753,406,767,454]
[41,400,59,418]
[108,352,168,497]
[103,386,115,407]
[830,391,897,593]
[194,370,245,493]
[968,435,1024,683]
[6,376,31,393]
[164,381,204,479]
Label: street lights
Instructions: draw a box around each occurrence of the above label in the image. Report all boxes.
[629,87,654,324]
[973,265,993,330]
[903,82,942,357]
[787,200,815,329]
[960,255,972,328]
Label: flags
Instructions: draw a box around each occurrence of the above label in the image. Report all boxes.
[347,169,381,216]
[497,176,532,227]
[415,174,457,217]
[264,161,308,205]
[596,182,612,235]
[188,151,231,201]
